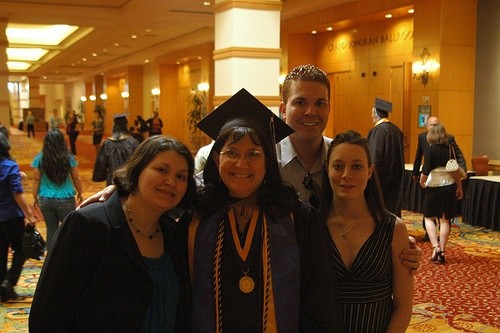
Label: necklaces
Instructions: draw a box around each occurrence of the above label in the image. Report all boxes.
[124,200,162,240]
[332,204,364,238]
[294,149,318,189]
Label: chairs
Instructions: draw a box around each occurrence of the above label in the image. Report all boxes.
[470,155,490,176]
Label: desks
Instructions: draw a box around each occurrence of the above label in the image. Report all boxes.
[402,164,500,231]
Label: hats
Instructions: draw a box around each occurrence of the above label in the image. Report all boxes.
[113,113,128,126]
[375,97,392,118]
[195,88,296,168]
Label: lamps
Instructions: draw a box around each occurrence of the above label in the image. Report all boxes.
[412,47,439,87]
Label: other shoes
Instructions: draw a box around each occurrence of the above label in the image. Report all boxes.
[0,280,15,297]
[422,232,429,241]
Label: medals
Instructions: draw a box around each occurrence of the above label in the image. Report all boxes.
[240,275,254,293]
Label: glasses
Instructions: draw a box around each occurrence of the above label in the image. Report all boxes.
[219,149,263,162]
[301,173,320,211]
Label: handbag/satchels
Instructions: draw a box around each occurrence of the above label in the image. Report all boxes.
[446,144,467,181]
[18,223,45,259]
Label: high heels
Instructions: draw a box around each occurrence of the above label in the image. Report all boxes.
[430,246,445,262]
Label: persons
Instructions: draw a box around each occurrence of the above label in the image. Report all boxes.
[28,134,197,333]
[301,128,416,333]
[0,132,37,302]
[364,98,404,219]
[93,114,140,186]
[193,64,338,212]
[411,114,468,264]
[31,128,85,251]
[74,116,423,333]
[20,103,164,156]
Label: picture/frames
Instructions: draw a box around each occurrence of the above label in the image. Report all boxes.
[417,104,431,129]
